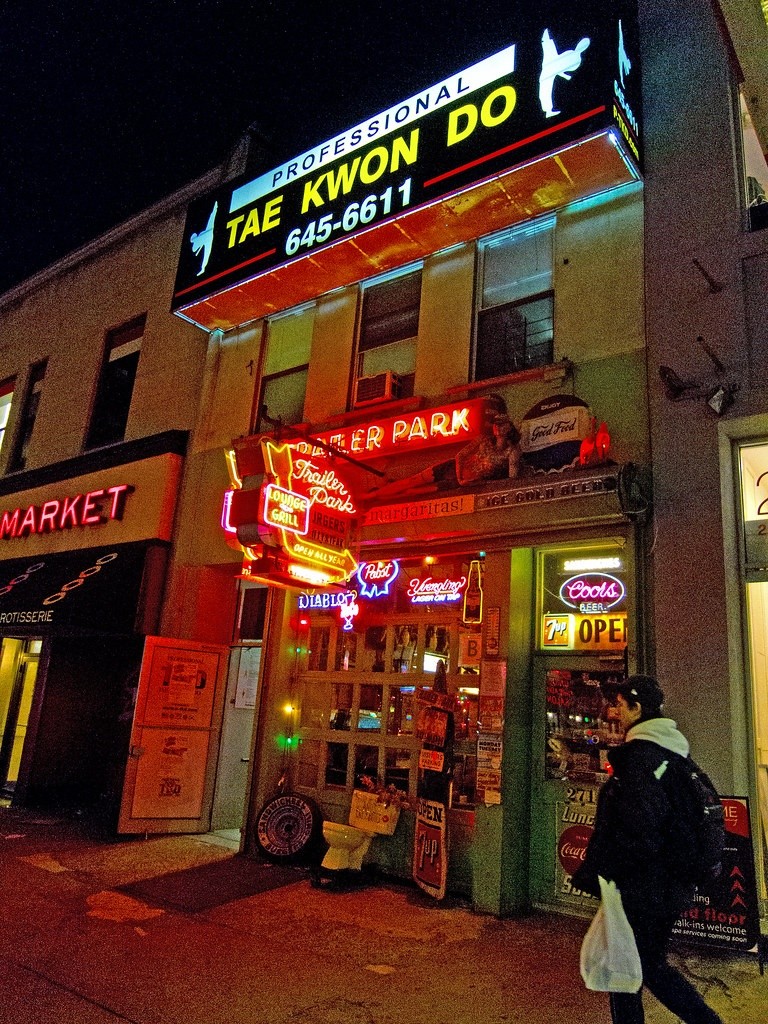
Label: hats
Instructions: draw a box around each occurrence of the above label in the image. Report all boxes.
[616,674,664,709]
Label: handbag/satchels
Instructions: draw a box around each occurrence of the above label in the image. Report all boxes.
[580,874,642,993]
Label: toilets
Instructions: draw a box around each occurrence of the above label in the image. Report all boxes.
[318,820,376,870]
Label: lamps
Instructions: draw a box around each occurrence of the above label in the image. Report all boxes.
[705,385,733,415]
[659,365,686,398]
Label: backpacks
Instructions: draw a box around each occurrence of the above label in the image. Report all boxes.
[665,749,727,879]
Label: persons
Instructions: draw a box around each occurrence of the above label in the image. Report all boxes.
[572,674,725,1024]
[360,414,522,504]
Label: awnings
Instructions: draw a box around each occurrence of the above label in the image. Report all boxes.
[0,545,146,640]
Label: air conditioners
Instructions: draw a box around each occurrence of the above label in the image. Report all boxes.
[352,370,403,408]
